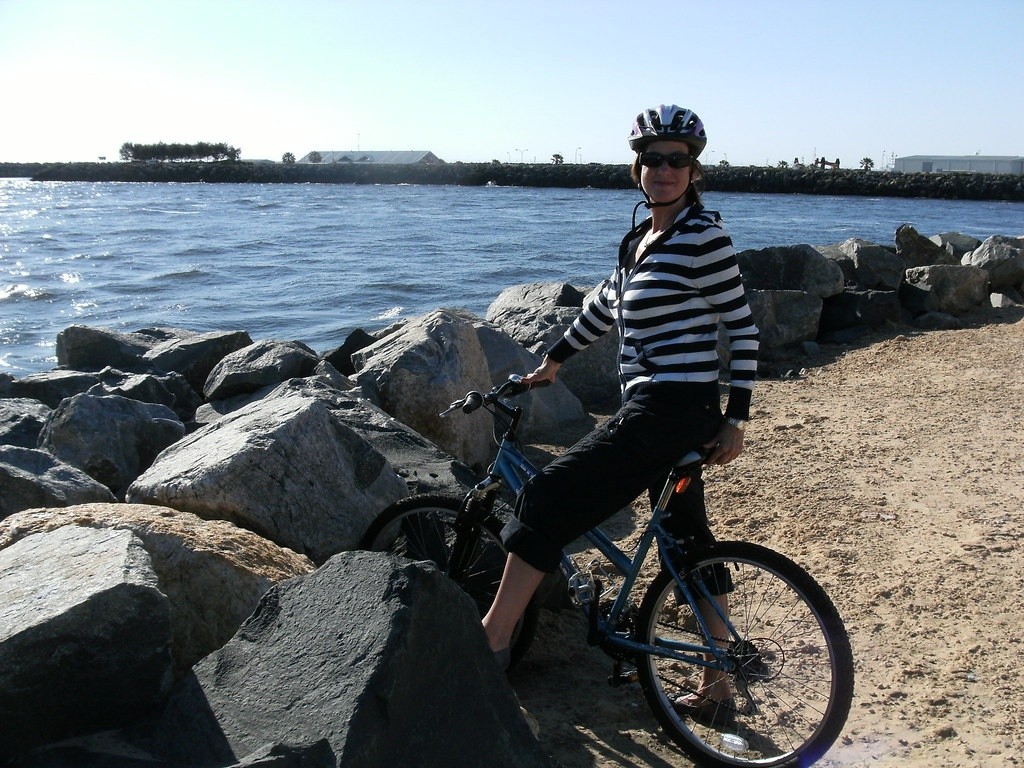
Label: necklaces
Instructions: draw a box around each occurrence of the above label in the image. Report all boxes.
[644,229,666,247]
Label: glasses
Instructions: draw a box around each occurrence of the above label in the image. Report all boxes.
[638,152,693,169]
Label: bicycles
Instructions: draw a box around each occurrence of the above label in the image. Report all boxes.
[356,374,855,768]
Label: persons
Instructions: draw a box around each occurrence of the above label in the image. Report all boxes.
[481,106,760,727]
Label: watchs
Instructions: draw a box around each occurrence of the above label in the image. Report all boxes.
[727,418,746,430]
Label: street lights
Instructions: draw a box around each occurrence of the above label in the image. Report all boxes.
[706,151,715,165]
[507,152,510,163]
[575,147,582,163]
[724,153,727,160]
[516,149,528,163]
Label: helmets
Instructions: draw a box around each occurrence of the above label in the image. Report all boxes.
[628,104,707,159]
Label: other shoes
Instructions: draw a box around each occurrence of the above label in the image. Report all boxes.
[493,647,511,673]
[664,691,737,725]
